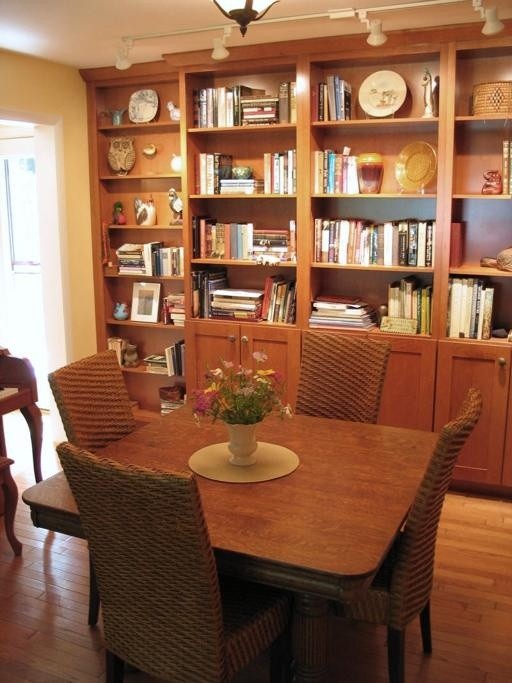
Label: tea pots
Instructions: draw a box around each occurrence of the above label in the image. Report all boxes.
[105,108,128,125]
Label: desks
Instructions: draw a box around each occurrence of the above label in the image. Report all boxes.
[23,402,442,681]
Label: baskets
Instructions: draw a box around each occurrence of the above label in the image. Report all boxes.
[472,81,512,115]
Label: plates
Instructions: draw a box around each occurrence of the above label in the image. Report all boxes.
[357,69,407,118]
[129,90,158,124]
[394,143,435,189]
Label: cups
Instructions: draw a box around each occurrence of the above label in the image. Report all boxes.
[358,152,383,193]
[233,167,253,180]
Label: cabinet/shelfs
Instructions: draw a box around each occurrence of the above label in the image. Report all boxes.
[300,49,449,432]
[84,72,185,421]
[433,44,512,496]
[186,65,298,413]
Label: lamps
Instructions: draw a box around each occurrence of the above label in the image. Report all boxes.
[356,10,388,47]
[212,0,281,38]
[471,0,505,35]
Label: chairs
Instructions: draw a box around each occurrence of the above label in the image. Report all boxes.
[290,386,485,679]
[46,351,138,452]
[51,440,294,683]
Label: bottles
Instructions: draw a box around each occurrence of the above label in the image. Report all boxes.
[161,298,170,324]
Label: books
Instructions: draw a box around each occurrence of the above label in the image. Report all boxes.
[500,139,510,195]
[162,291,186,326]
[308,275,496,341]
[190,81,298,326]
[115,241,184,277]
[508,140,512,196]
[450,223,463,266]
[107,338,186,376]
[154,386,185,415]
[311,74,359,196]
[313,217,433,267]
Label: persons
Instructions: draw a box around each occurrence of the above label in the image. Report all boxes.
[418,66,435,118]
